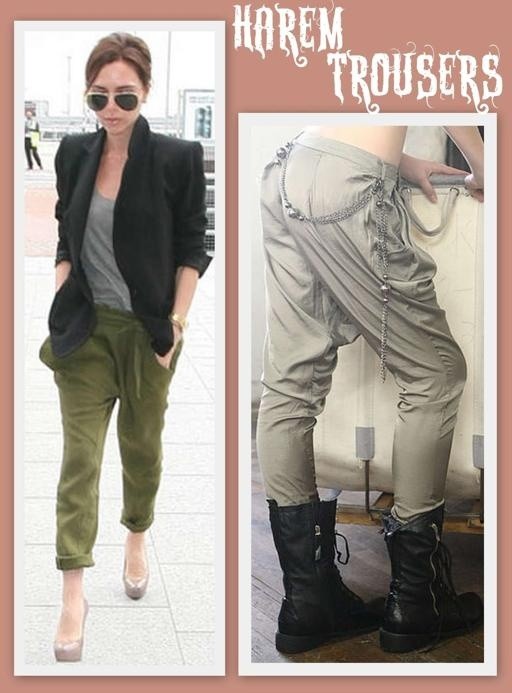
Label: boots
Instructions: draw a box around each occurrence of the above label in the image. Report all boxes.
[375,501,484,655]
[260,494,386,658]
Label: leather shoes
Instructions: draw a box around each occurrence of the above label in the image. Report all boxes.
[120,540,155,600]
[51,594,92,665]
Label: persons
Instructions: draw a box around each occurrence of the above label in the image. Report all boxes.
[37,30,214,663]
[23,110,45,170]
[258,124,484,654]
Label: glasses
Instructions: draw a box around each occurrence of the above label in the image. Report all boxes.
[80,84,147,116]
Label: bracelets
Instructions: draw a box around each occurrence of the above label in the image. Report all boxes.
[168,312,189,330]
